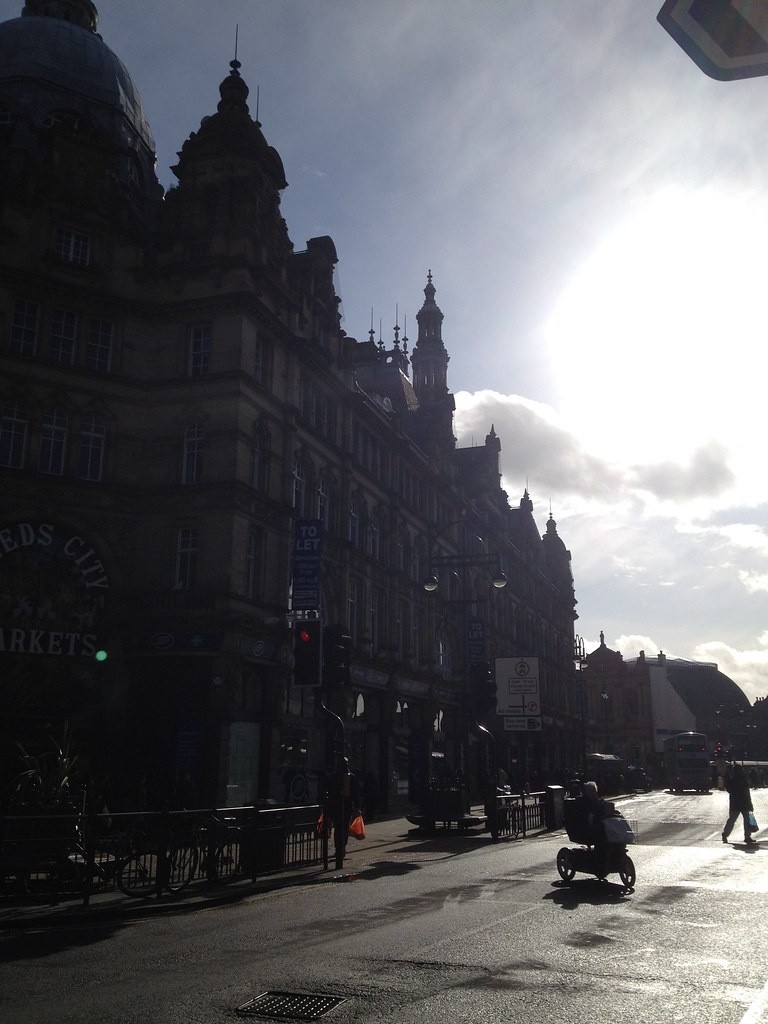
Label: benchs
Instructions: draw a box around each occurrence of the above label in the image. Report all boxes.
[406,814,488,830]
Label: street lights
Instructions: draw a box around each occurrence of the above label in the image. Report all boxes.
[573,640,588,766]
[423,519,509,815]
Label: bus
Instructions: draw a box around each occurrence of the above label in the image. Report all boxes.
[664,732,713,793]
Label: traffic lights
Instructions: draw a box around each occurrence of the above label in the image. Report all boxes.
[326,625,352,685]
[290,618,323,687]
[717,745,723,757]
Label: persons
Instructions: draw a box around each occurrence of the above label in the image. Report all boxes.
[749,764,768,790]
[573,780,628,874]
[496,760,508,788]
[323,756,362,870]
[722,763,756,843]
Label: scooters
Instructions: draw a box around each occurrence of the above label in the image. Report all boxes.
[557,798,635,887]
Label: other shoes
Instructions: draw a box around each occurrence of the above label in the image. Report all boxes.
[722,833,727,843]
[744,837,756,842]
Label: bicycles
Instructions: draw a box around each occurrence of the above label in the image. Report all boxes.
[19,813,171,904]
[160,812,245,895]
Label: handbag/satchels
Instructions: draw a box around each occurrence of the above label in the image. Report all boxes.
[315,813,331,839]
[350,815,366,840]
[746,812,759,832]
